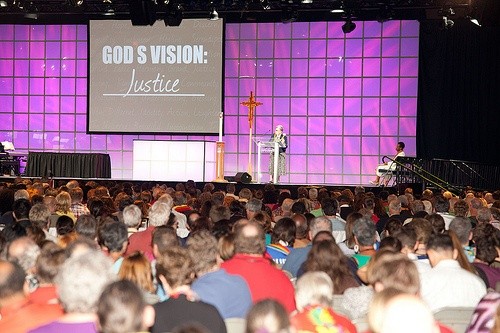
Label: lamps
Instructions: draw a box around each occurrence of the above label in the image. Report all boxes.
[129,1,157,26]
[164,0,184,27]
[341,16,358,34]
[24,0,39,20]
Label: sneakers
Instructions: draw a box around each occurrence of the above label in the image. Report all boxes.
[369,180,378,186]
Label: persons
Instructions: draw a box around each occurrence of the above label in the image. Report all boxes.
[219,222,297,317]
[351,218,377,270]
[449,215,476,263]
[368,142,405,187]
[320,198,345,245]
[269,125,288,184]
[264,217,297,266]
[0,177,500,333]
[27,248,116,333]
[288,271,358,333]
[291,213,311,248]
[0,260,65,333]
[246,298,297,333]
[122,201,170,262]
[145,246,226,333]
[185,230,252,318]
[96,278,155,333]
[419,234,487,333]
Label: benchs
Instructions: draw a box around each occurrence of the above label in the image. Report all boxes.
[379,156,424,183]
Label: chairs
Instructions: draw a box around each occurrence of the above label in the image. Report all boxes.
[0,141,23,177]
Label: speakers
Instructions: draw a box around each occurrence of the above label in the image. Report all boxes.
[235,172,252,183]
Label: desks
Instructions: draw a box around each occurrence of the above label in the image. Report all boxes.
[26,151,111,178]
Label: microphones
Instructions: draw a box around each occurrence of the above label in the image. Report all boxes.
[261,130,270,137]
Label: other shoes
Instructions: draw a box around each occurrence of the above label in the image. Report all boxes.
[269,180,280,184]
